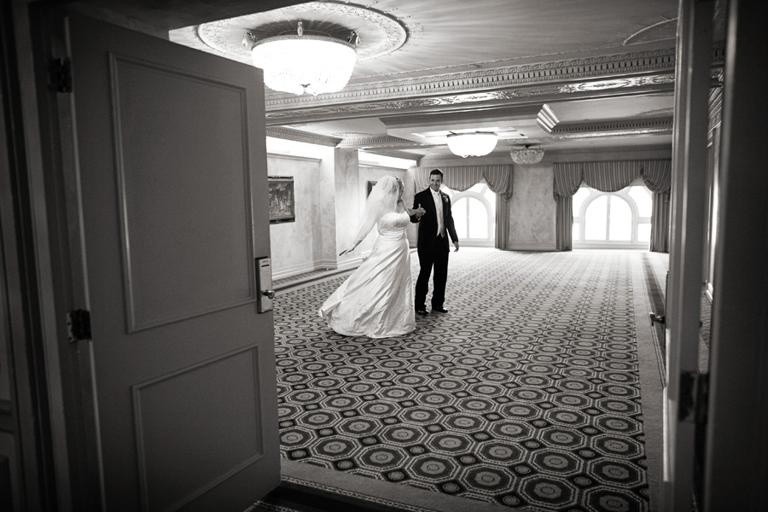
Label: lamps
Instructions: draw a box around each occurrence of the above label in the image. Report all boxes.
[508,137,545,168]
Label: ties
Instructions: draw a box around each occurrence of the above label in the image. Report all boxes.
[435,192,445,238]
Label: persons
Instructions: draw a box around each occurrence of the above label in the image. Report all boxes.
[339,168,459,328]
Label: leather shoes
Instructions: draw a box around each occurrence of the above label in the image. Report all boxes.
[432,307,448,313]
[417,309,429,314]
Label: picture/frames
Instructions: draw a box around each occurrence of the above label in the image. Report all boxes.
[264,175,296,225]
